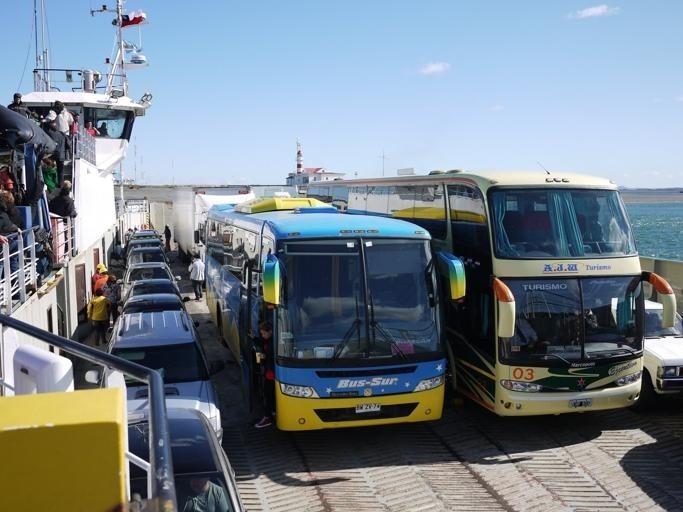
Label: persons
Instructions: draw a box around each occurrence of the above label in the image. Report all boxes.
[85,288,111,347]
[247,320,275,430]
[187,254,204,300]
[0,93,108,300]
[502,317,551,353]
[101,274,121,334]
[161,225,171,251]
[176,476,229,511]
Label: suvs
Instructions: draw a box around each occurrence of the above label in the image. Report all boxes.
[85,309,224,446]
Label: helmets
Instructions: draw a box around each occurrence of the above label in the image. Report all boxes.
[99,268,108,273]
[97,263,106,269]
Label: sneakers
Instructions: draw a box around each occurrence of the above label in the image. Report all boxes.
[254,417,273,428]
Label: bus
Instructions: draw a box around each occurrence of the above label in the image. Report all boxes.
[308,168,678,415]
[195,196,466,432]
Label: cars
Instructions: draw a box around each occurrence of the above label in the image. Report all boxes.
[116,229,190,315]
[127,408,243,511]
[610,295,683,414]
